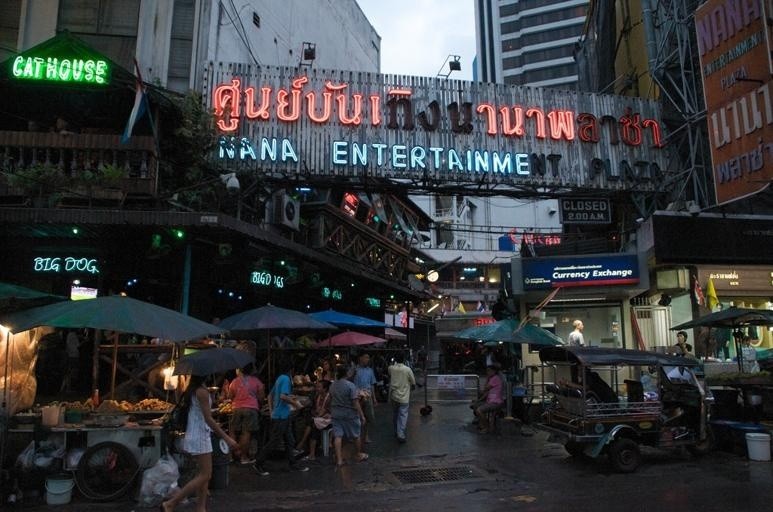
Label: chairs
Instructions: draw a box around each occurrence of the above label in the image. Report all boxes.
[486,383,607,427]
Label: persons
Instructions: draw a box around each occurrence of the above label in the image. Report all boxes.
[161,360,239,511]
[27,106,96,177]
[568,320,585,384]
[42,329,159,397]
[672,331,691,356]
[220,344,427,475]
[446,341,509,434]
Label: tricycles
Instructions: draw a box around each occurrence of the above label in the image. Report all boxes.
[42,426,168,502]
[537,340,716,470]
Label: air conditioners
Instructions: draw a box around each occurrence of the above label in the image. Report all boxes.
[271,192,300,232]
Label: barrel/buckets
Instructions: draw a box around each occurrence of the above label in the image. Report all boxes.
[708,417,759,452]
[43,468,76,506]
[745,432,772,462]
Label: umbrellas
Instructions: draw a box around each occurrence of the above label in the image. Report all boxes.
[215,302,338,392]
[669,304,773,371]
[1,282,67,301]
[452,317,565,417]
[3,292,229,396]
[307,307,390,360]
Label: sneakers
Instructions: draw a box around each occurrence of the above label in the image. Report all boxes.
[289,448,316,473]
[240,456,256,465]
[253,464,269,476]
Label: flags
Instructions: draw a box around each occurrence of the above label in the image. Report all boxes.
[121,82,146,144]
[694,280,704,306]
[706,278,719,311]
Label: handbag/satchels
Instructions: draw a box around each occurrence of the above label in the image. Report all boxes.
[163,391,190,441]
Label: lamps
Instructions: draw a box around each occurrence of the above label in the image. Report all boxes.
[297,41,462,80]
